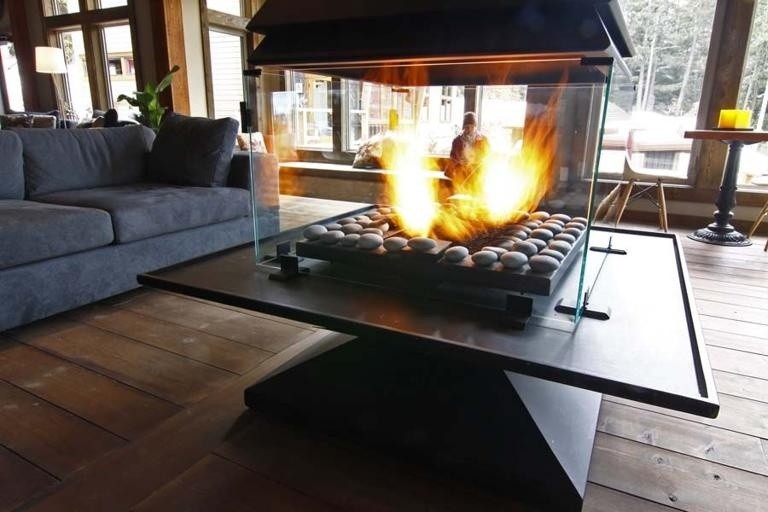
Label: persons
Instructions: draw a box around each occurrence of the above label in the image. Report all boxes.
[449,111,489,192]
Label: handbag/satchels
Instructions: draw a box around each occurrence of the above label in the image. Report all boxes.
[445,163,470,183]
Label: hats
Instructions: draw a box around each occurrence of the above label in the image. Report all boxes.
[463,111,476,124]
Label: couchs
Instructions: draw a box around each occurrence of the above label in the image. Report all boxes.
[0,127,278,334]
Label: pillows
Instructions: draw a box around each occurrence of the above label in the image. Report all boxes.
[146,112,238,189]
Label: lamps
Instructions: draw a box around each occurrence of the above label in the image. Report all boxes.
[33,47,69,127]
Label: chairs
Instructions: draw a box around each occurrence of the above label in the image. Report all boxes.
[593,128,674,232]
[1,108,124,126]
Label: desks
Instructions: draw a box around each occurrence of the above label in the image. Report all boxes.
[683,129,768,246]
